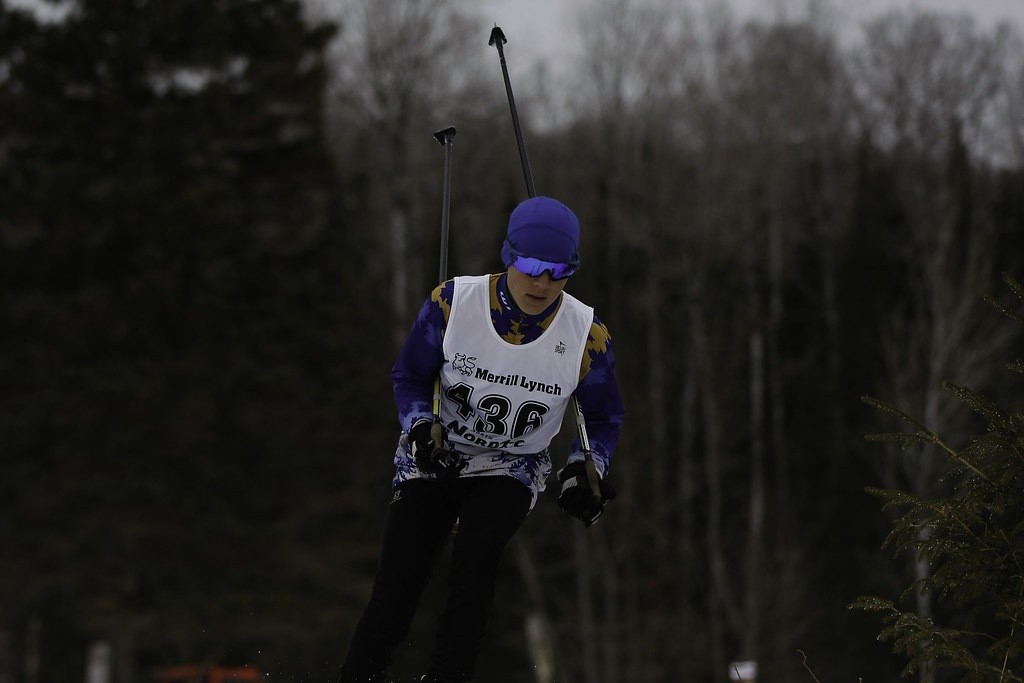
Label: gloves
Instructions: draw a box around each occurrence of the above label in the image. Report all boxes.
[407,417,447,473]
[557,464,615,527]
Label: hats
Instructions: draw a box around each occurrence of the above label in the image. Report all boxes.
[501,196,580,277]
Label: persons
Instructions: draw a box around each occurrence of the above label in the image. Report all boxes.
[334,196,622,683]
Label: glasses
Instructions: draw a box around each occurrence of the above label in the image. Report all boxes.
[510,248,581,282]
